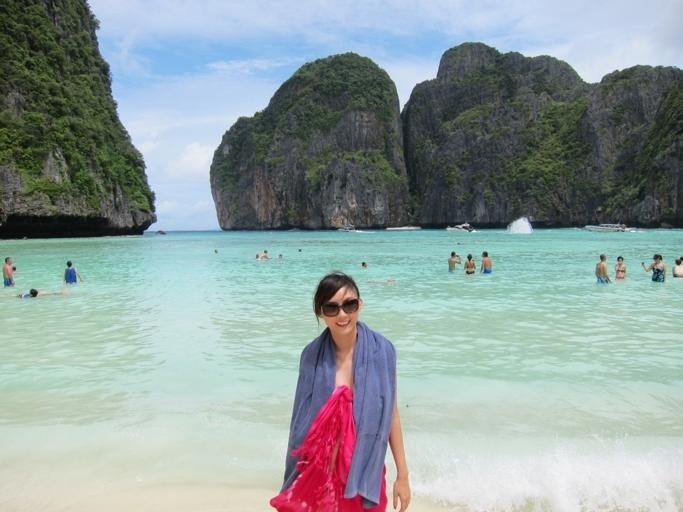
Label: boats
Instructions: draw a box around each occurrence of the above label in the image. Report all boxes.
[584,219,630,234]
[338,222,358,233]
[388,223,423,231]
[443,219,478,234]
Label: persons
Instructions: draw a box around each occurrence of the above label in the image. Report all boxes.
[671,257,683,279]
[1,257,16,289]
[268,270,412,512]
[360,261,367,268]
[62,258,83,283]
[447,251,461,272]
[16,289,52,299]
[479,252,491,274]
[254,250,284,260]
[463,254,476,274]
[594,254,612,285]
[642,254,668,284]
[614,256,627,280]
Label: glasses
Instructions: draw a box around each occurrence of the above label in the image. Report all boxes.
[321,300,359,317]
